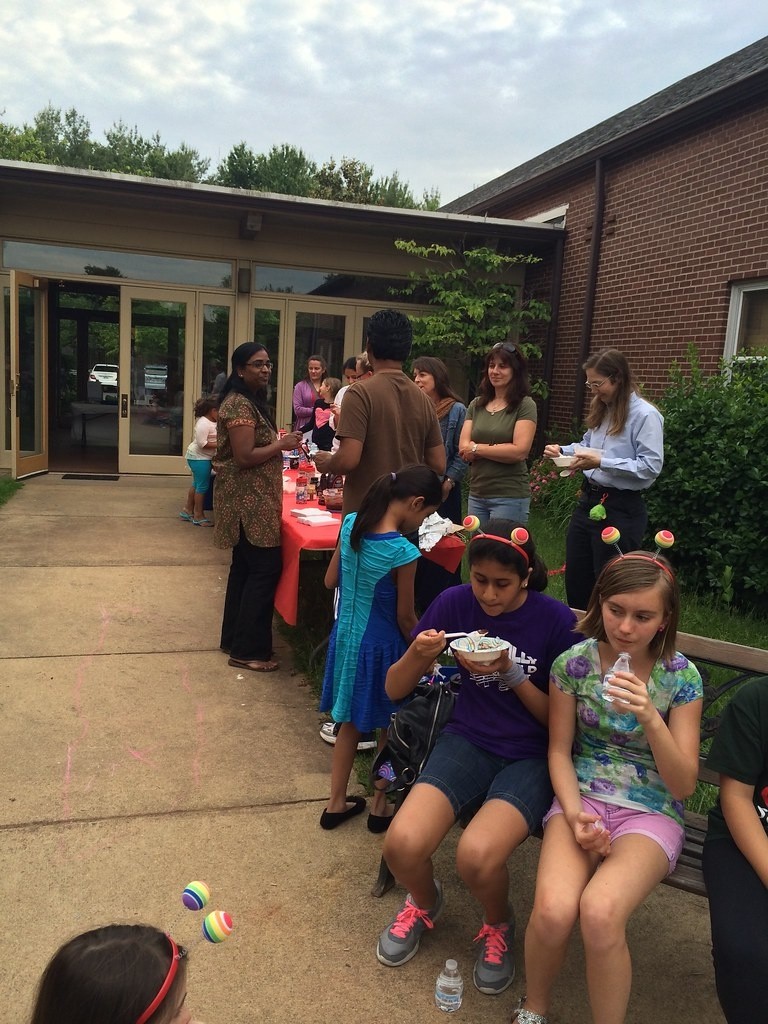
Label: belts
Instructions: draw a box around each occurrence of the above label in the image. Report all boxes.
[585,484,640,496]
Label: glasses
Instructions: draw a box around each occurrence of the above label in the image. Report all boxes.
[356,370,372,380]
[246,362,273,369]
[586,374,613,390]
[493,342,516,354]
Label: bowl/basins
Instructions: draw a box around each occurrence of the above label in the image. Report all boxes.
[549,456,576,467]
[450,637,510,666]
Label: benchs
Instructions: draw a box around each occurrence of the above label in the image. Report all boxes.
[372,603,768,900]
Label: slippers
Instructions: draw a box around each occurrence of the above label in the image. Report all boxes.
[228,658,279,672]
[179,512,194,521]
[193,518,215,527]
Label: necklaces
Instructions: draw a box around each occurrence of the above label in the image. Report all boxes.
[490,401,504,416]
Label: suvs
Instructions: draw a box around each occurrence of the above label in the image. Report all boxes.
[142,364,168,391]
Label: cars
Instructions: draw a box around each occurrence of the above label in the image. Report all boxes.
[88,364,118,391]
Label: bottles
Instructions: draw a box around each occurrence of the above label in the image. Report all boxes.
[309,477,319,499]
[318,473,343,506]
[602,652,631,713]
[295,477,308,504]
[278,428,286,440]
[434,960,464,1012]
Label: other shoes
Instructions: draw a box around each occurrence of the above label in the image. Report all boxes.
[319,796,367,829]
[368,803,394,834]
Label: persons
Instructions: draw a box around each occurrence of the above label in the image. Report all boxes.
[30,925,205,1024]
[314,309,446,752]
[458,341,537,536]
[509,550,704,1024]
[378,520,584,995]
[209,359,227,400]
[179,399,221,527]
[412,357,467,580]
[293,351,375,486]
[211,340,303,673]
[544,347,664,612]
[319,465,442,834]
[702,675,768,1024]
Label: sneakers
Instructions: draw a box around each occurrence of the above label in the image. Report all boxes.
[319,721,377,750]
[377,879,445,966]
[473,902,516,994]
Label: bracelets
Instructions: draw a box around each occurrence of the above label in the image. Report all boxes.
[447,477,455,488]
[499,660,527,689]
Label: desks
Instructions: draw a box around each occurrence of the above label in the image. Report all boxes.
[273,448,466,664]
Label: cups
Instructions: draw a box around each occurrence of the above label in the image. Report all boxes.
[290,456,299,470]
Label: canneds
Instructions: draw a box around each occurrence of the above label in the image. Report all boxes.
[290,457,299,469]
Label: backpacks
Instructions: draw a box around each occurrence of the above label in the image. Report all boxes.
[371,682,454,791]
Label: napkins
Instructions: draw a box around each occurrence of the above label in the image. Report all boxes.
[291,507,339,528]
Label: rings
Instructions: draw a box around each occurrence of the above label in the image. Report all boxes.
[297,435,300,438]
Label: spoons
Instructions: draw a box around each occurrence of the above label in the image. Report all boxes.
[560,468,580,477]
[444,628,490,638]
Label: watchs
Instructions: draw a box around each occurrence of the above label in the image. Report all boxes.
[471,444,479,457]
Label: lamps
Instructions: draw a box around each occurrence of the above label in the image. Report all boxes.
[240,214,262,240]
